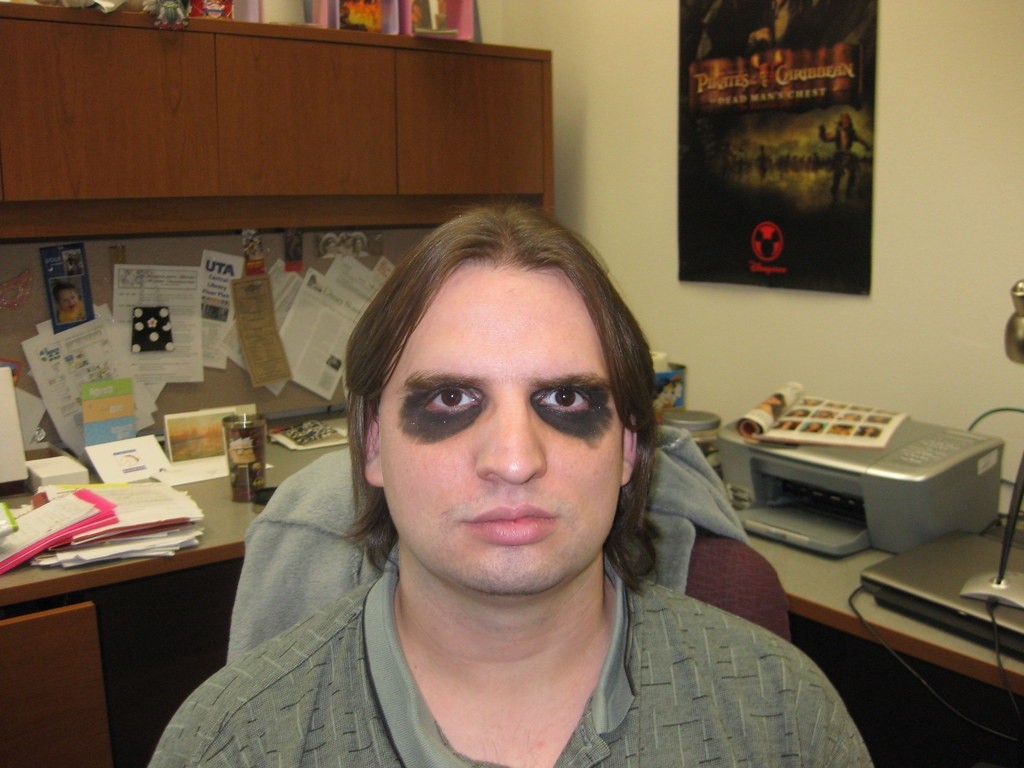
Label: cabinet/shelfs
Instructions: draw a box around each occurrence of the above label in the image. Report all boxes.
[0,2,554,243]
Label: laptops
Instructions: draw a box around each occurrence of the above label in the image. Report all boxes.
[859,528,1024,662]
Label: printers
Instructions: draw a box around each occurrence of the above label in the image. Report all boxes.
[717,411,1003,556]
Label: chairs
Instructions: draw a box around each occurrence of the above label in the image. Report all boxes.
[638,523,791,643]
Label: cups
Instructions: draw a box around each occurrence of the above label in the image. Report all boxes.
[222,414,267,502]
[650,363,687,422]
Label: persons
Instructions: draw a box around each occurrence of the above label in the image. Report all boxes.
[54,281,86,325]
[144,202,877,768]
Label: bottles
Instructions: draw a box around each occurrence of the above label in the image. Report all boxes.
[660,409,723,478]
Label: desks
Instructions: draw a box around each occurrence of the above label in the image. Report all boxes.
[0,413,1024,768]
